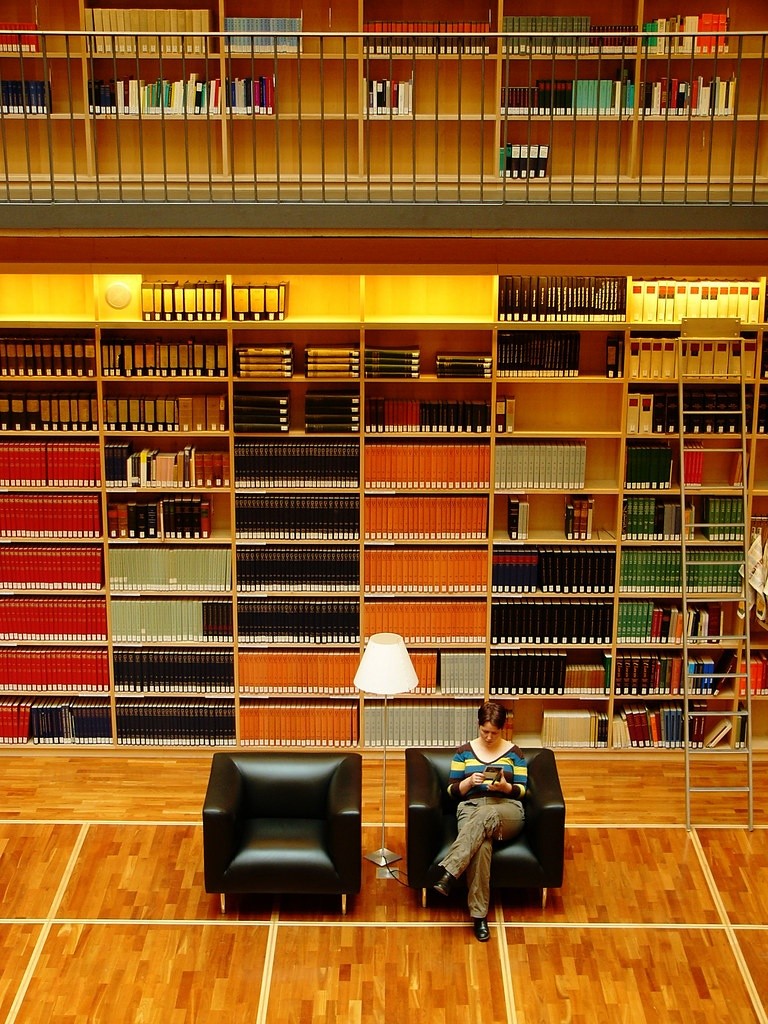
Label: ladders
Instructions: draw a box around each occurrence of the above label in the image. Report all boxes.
[676,337,755,832]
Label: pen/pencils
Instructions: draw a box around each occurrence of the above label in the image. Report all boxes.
[486,779,497,792]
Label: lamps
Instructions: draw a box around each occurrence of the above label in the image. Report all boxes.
[353,634,418,868]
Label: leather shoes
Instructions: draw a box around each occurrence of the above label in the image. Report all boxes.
[434,871,453,896]
[473,915,490,940]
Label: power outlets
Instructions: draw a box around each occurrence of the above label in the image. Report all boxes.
[376,868,400,880]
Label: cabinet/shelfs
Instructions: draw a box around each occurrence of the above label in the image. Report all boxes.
[0,0,768,184]
[0,275,768,755]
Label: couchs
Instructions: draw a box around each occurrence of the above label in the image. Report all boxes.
[201,752,363,914]
[404,746,566,909]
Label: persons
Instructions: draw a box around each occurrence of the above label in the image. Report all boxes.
[432,701,528,939]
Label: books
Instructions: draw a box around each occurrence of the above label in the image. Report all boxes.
[0,79,52,114]
[226,75,275,114]
[142,279,290,321]
[496,275,626,322]
[88,72,223,115]
[640,7,730,55]
[501,68,634,116]
[639,75,737,117]
[84,8,214,53]
[499,141,549,178]
[0,23,42,53]
[501,16,636,55]
[362,328,768,749]
[631,277,760,325]
[223,17,302,53]
[0,333,360,746]
[362,72,413,117]
[363,20,489,55]
[483,766,504,785]
